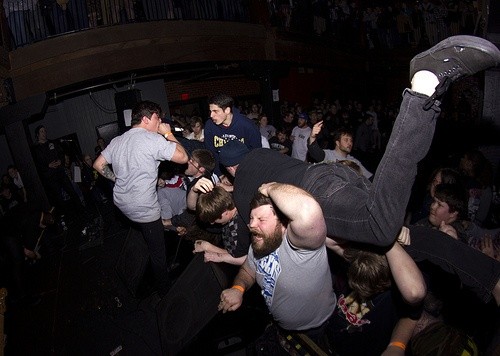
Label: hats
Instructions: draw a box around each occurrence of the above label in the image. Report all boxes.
[296,113,309,119]
[220,140,251,166]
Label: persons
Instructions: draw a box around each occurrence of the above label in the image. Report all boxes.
[217,34,500,247]
[0,91,500,356]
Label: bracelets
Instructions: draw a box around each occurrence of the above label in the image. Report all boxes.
[387,343,407,352]
[310,132,317,138]
[164,131,173,139]
[231,284,246,293]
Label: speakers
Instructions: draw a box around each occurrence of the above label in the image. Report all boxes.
[155,252,271,356]
[117,222,196,297]
[115,88,142,135]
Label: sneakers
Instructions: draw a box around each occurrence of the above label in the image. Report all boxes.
[408,35,500,111]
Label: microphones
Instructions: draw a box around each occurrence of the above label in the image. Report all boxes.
[171,127,184,132]
[60,139,75,142]
